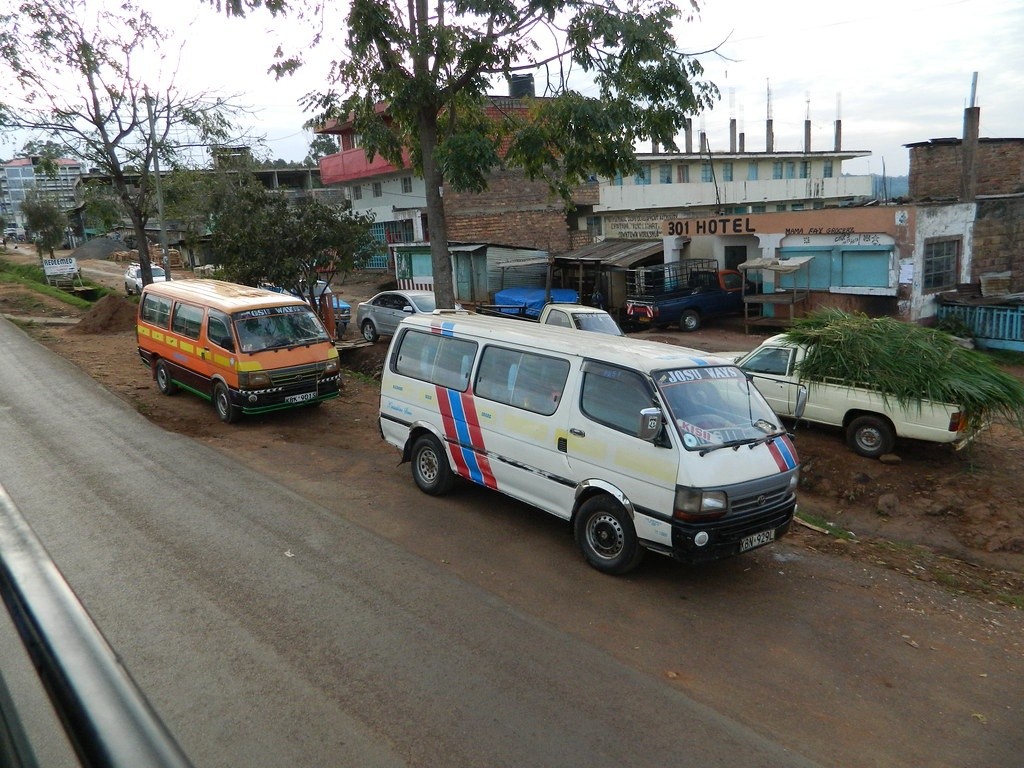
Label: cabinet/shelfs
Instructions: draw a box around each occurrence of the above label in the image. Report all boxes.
[737,255,816,335]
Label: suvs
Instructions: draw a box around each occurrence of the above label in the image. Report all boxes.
[125,262,174,295]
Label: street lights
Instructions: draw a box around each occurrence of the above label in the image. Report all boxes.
[147,97,171,281]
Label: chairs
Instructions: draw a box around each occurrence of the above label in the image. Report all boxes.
[460,355,492,399]
[506,363,547,413]
[420,347,450,386]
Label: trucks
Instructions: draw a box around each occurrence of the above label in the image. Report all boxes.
[622,257,760,332]
[474,302,629,384]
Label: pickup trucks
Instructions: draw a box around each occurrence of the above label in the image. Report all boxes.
[7,234,24,242]
[257,280,351,330]
[692,331,996,458]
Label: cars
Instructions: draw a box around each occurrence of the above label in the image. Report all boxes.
[357,288,470,342]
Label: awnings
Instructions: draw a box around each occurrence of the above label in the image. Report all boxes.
[496,256,551,303]
[448,244,485,252]
[554,238,691,304]
[737,256,814,297]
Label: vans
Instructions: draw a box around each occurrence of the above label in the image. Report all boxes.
[136,278,341,423]
[378,308,800,574]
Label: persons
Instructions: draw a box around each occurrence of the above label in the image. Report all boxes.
[591,286,604,309]
